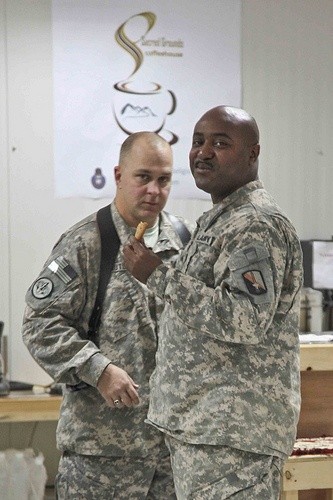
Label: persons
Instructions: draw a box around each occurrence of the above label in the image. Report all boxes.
[118,104,304,500]
[22,130,199,500]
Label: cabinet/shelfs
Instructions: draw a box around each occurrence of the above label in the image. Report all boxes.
[261,326,333,499]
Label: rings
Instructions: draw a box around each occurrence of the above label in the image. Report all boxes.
[113,399,120,407]
